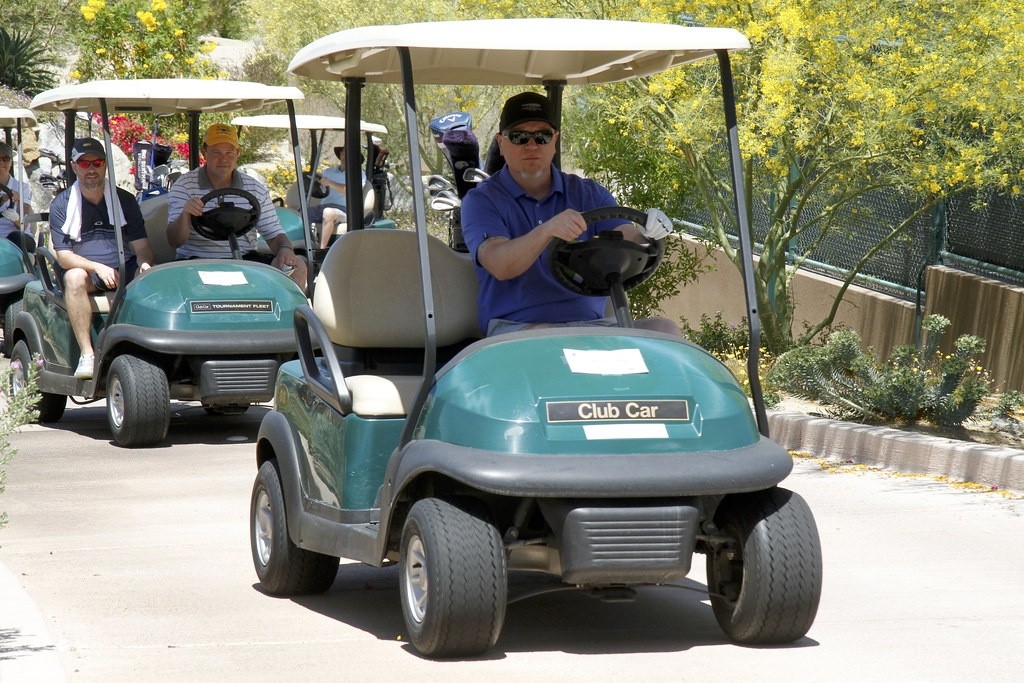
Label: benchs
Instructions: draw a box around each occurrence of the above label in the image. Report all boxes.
[287,176,375,220]
[294,228,684,414]
[34,195,174,315]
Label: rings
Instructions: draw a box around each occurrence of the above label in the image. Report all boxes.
[288,261,293,263]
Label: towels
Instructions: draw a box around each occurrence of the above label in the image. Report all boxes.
[60,178,127,242]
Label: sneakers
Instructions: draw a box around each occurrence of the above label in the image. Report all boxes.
[74,354,95,379]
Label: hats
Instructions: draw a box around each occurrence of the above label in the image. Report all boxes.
[499,92,558,129]
[334,145,365,166]
[70,138,107,163]
[203,124,241,149]
[0,142,16,158]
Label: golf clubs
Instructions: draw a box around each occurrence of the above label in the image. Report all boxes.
[145,163,183,192]
[39,170,68,195]
[362,135,389,172]
[426,168,492,212]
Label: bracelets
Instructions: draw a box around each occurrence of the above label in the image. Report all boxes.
[1,194,8,202]
[279,246,294,250]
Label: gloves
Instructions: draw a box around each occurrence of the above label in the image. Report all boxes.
[634,208,673,241]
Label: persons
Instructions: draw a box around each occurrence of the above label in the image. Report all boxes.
[302,146,366,250]
[49,138,157,379]
[0,142,36,254]
[460,91,673,338]
[166,124,307,293]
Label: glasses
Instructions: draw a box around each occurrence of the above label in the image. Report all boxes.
[76,158,106,168]
[499,129,554,145]
[0,156,11,162]
